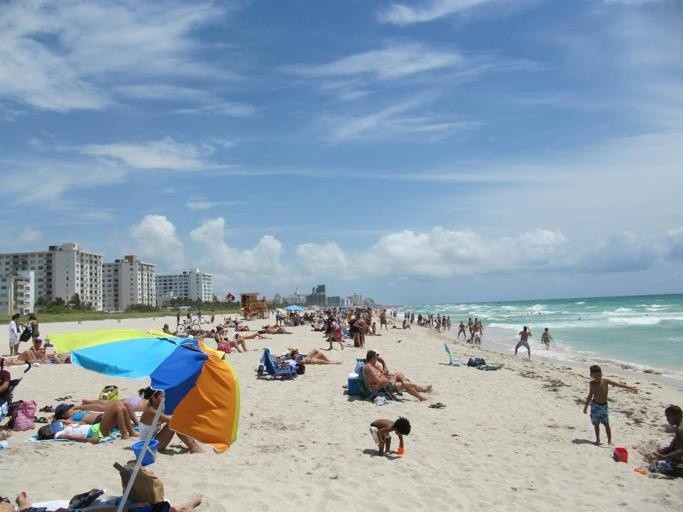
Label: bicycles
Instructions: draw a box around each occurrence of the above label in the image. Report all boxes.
[175,318,201,335]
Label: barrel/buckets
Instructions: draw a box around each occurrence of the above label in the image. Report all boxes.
[613,447,628,461]
[130,439,161,467]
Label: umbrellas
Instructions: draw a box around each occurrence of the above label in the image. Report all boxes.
[45,328,240,512]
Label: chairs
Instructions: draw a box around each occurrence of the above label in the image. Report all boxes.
[176,315,209,327]
[357,358,403,402]
[443,343,477,362]
[0,360,31,407]
[161,327,189,339]
[262,346,297,381]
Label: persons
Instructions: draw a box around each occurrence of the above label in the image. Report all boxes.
[139,386,204,453]
[582,365,638,445]
[540,326,553,350]
[0,312,139,453]
[76,398,151,413]
[359,349,435,402]
[643,406,683,475]
[369,419,411,456]
[161,305,544,379]
[0,491,203,512]
